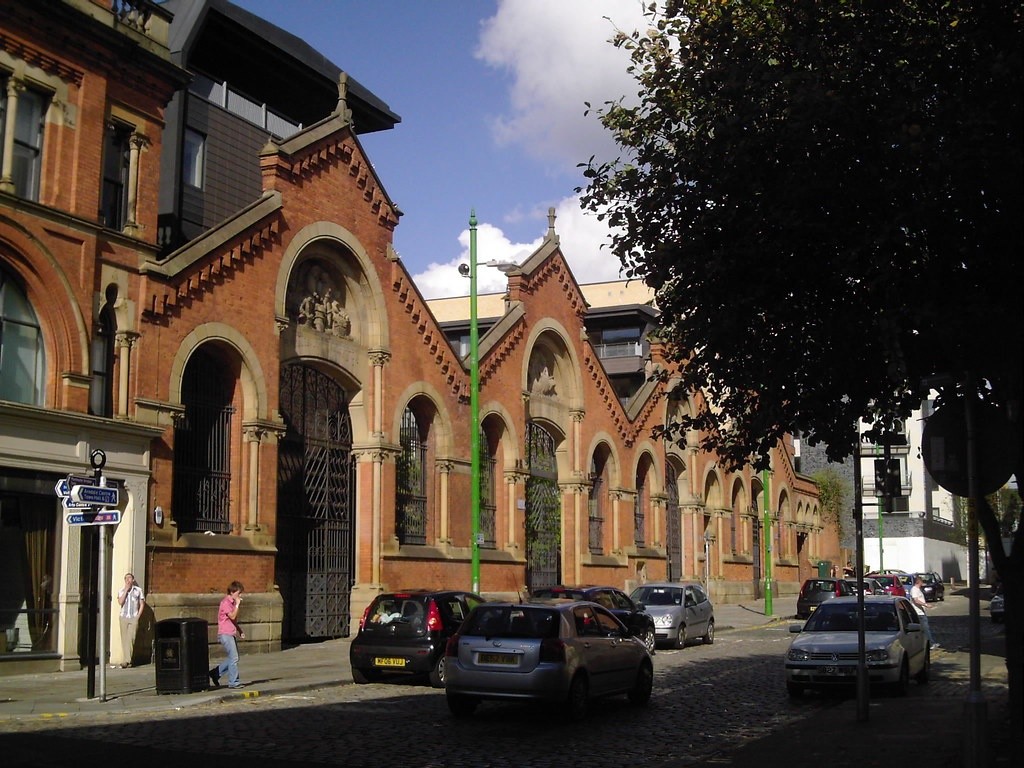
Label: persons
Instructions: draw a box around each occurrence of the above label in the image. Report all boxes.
[843,561,854,578]
[208,581,247,689]
[116,572,146,668]
[909,577,941,650]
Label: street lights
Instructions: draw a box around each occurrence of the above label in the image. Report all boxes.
[470,262,522,596]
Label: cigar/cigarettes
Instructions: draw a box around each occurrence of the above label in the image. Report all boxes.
[239,598,243,601]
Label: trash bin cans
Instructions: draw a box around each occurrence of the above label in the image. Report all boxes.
[154,617,210,695]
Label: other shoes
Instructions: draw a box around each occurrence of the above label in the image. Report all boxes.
[930,643,940,649]
[127,663,132,668]
[208,668,220,687]
[119,662,128,668]
[228,683,245,689]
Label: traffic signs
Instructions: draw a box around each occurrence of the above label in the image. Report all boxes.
[54,473,120,526]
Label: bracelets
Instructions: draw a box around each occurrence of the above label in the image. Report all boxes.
[925,603,927,607]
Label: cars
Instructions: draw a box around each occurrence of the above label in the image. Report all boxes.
[350,590,486,688]
[628,581,715,650]
[531,584,656,653]
[897,574,915,600]
[866,574,906,597]
[843,578,883,596]
[990,586,1005,623]
[784,595,930,698]
[914,571,945,602]
[444,599,654,723]
[797,579,856,616]
